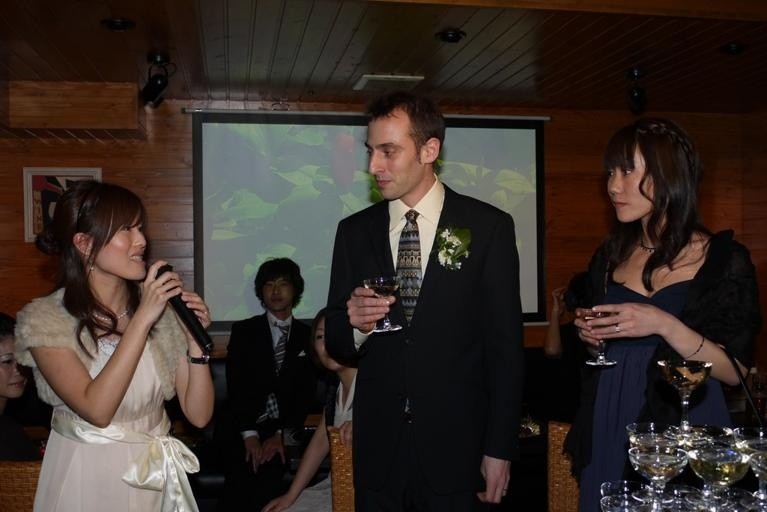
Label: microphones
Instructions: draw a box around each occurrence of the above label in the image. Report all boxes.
[145,260,214,360]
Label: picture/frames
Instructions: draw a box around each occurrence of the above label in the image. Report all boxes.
[22,166,101,243]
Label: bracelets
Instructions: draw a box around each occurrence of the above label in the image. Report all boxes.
[684,336,705,360]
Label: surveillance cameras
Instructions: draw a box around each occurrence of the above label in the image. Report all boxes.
[137,54,177,109]
[626,86,647,116]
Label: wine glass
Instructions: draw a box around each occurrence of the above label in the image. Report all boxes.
[663,360,713,435]
[363,278,403,333]
[600,422,767,512]
[578,308,618,366]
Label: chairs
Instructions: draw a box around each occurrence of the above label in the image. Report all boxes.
[327,427,355,512]
[548,422,580,512]
[0,461,43,512]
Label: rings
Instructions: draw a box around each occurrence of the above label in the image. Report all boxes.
[503,488,508,491]
[615,323,623,333]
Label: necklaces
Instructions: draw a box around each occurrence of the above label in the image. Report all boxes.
[92,307,133,324]
[634,229,679,254]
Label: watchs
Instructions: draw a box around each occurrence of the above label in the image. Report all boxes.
[182,348,213,365]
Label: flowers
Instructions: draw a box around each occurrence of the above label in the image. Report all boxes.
[437,229,473,271]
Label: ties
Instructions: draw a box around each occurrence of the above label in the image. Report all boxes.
[266,325,289,420]
[396,210,423,326]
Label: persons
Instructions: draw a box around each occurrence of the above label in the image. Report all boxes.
[225,258,332,511]
[261,306,356,511]
[547,273,593,376]
[14,182,213,512]
[576,117,758,511]
[324,92,523,512]
[0,313,44,463]
